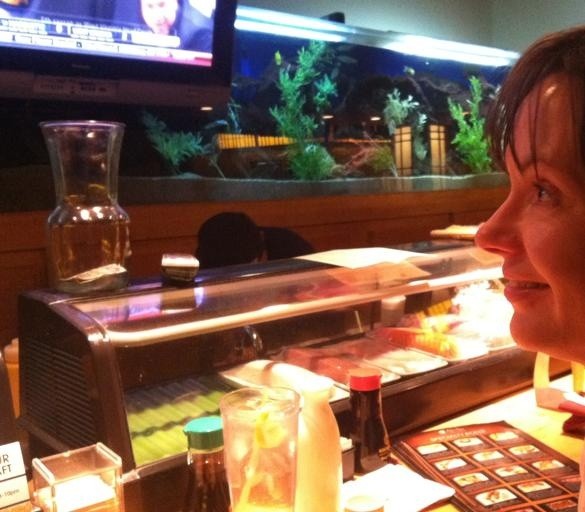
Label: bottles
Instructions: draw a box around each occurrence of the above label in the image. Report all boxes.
[347,367,391,477]
[183,413,231,512]
[295,382,344,512]
[38,119,132,298]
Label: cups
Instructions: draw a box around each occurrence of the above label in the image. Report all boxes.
[219,386,297,512]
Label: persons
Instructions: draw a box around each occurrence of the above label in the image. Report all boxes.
[140,1,214,53]
[474,26,585,365]
[195,211,317,268]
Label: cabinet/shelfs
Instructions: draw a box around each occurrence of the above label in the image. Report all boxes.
[18,238,572,512]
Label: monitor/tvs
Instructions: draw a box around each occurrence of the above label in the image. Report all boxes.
[0,0,236,107]
